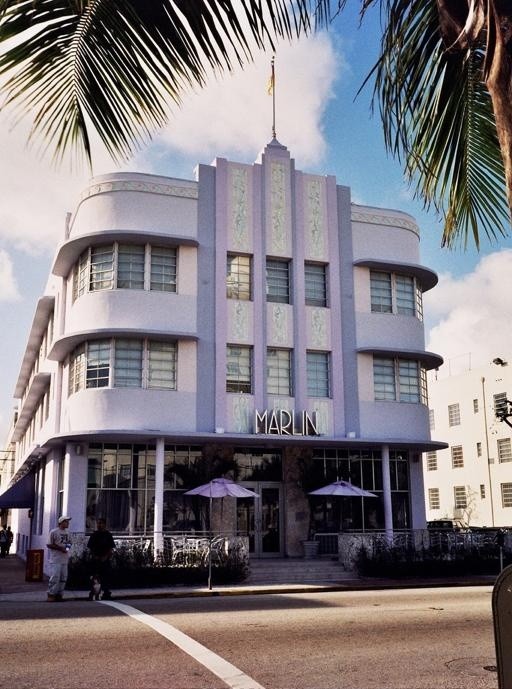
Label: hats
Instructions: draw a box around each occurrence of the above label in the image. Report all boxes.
[56,515,72,525]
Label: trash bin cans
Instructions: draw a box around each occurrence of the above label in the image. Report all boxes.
[25,549,44,582]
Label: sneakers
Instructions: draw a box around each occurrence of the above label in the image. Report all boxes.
[45,591,66,602]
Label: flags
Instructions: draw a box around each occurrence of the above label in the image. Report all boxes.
[266,67,274,96]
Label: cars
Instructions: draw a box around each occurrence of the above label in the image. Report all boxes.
[426,518,512,561]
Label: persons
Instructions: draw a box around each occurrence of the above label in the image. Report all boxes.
[5,527,13,555]
[0,524,9,559]
[87,518,117,601]
[46,516,72,601]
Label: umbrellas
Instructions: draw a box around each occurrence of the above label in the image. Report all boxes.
[307,480,379,532]
[183,474,261,538]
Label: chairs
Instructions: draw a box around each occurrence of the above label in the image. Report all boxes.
[112,536,252,565]
[381,530,496,551]
